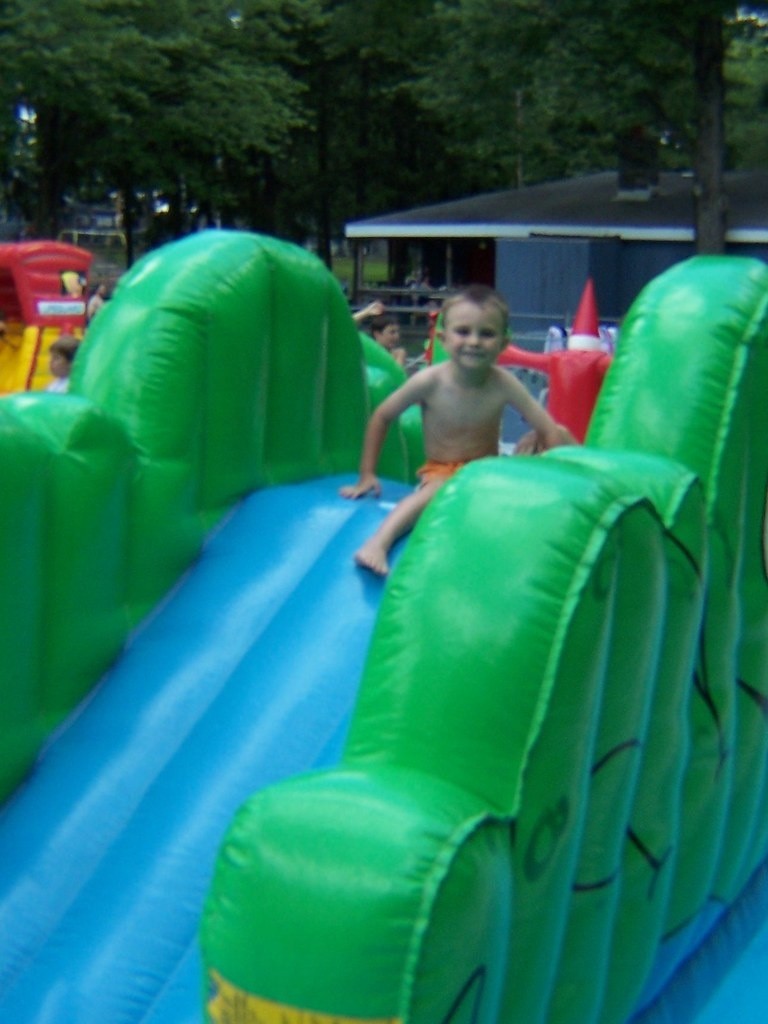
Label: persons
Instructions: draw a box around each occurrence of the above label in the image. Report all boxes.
[420,276,434,291]
[337,285,581,578]
[85,284,108,327]
[47,336,82,395]
